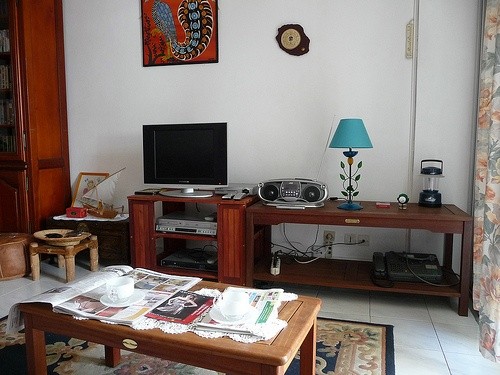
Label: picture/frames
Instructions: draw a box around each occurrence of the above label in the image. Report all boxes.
[141,0,221,68]
[71,171,110,206]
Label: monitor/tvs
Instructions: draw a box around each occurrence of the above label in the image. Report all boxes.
[142,122,227,198]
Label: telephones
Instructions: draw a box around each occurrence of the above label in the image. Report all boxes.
[372,251,442,283]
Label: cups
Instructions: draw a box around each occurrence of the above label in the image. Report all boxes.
[218,292,249,321]
[106,277,135,303]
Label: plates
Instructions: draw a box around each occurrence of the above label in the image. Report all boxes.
[209,304,257,325]
[34,229,91,246]
[100,289,145,308]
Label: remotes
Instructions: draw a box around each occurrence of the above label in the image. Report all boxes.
[233,193,246,199]
[207,255,218,264]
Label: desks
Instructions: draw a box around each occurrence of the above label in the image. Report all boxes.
[17,265,322,375]
[245,199,474,317]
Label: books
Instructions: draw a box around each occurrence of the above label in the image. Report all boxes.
[145,290,216,325]
[5,265,201,334]
[0,30,10,52]
[0,100,13,124]
[0,65,12,88]
[0,136,16,152]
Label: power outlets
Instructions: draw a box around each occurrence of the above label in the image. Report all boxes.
[344,233,357,244]
[359,234,370,246]
[324,245,335,257]
[323,232,336,243]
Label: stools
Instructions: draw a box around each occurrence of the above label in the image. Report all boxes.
[30,233,98,283]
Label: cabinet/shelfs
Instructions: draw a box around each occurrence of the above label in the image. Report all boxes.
[126,190,252,285]
[0,0,72,234]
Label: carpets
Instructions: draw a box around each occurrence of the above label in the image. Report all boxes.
[0,307,397,375]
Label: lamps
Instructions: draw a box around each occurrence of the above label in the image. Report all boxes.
[326,115,375,210]
[418,157,446,206]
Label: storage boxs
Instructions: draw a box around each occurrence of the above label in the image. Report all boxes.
[48,216,131,266]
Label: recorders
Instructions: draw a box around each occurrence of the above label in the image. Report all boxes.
[258,177,328,204]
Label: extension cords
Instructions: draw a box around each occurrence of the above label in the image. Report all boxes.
[270,257,280,276]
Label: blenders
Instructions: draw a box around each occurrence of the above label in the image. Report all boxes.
[419,160,446,208]
